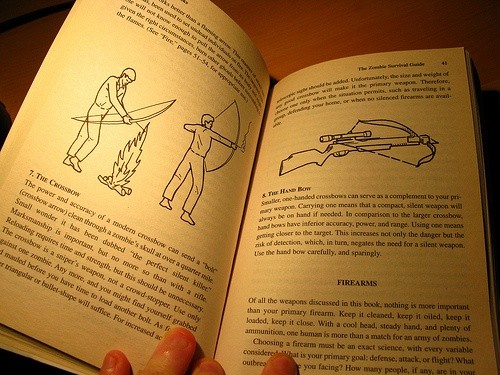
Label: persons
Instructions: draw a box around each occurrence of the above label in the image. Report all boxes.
[98,327,299,375]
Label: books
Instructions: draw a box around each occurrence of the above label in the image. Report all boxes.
[0,0,500,375]
